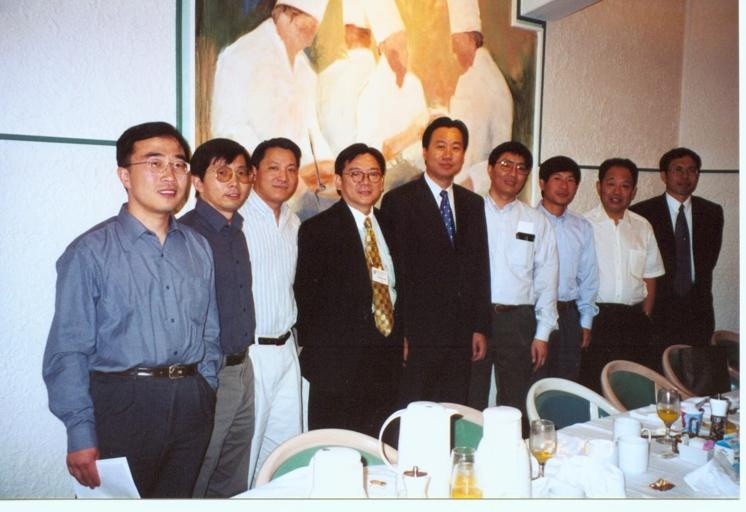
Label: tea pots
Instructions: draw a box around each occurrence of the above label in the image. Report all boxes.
[377,399,456,499]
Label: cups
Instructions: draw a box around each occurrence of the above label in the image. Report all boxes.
[447,445,482,499]
[587,417,651,474]
[681,404,705,436]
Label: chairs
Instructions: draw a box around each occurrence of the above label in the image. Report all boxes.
[257,428,396,484]
[525,378,619,431]
[601,359,686,412]
[710,331,739,377]
[379,402,488,470]
[662,343,737,395]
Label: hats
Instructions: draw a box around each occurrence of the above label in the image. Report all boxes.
[276,0,328,23]
[342,1,371,30]
[448,0,482,35]
[366,1,404,45]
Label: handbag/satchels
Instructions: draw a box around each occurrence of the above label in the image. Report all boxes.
[680,346,730,396]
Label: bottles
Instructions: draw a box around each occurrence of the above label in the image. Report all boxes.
[474,406,530,499]
[710,397,729,441]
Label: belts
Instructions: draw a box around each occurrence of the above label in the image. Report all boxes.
[222,350,245,365]
[491,303,518,314]
[252,331,291,345]
[98,363,197,380]
[556,301,572,311]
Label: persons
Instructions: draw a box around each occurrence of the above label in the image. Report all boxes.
[237,138,302,490]
[629,148,724,359]
[42,121,222,499]
[380,117,491,448]
[293,143,405,445]
[529,156,599,383]
[580,159,666,377]
[176,139,255,496]
[468,142,560,440]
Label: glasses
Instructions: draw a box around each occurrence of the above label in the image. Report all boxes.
[495,160,529,175]
[341,171,382,183]
[205,166,254,183]
[124,158,191,175]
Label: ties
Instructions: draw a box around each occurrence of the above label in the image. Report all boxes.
[440,190,455,250]
[364,218,395,338]
[673,204,692,298]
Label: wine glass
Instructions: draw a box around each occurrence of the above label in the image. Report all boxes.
[656,388,681,444]
[528,419,557,482]
